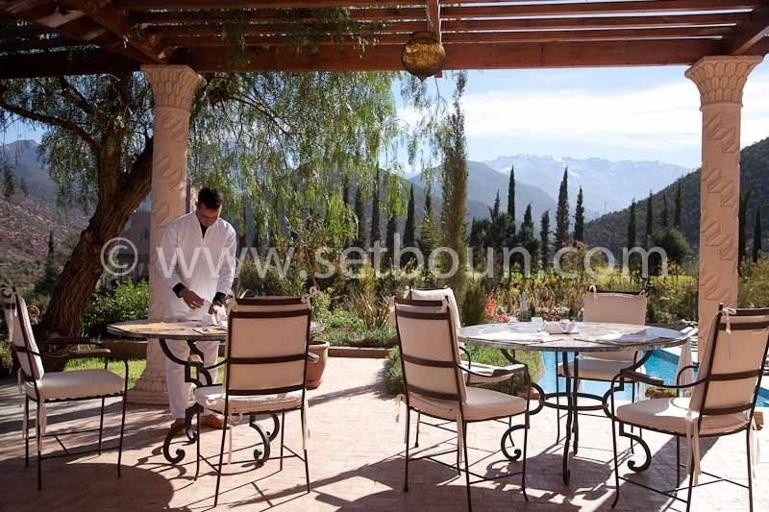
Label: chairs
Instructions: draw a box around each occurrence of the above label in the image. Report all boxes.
[0,284,126,492]
[388,283,769,512]
[193,295,311,508]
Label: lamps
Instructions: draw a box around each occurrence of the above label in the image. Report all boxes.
[400,0,446,85]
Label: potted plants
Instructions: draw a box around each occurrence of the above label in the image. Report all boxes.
[240,261,334,389]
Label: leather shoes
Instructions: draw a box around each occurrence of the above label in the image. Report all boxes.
[171,418,186,435]
[201,412,225,428]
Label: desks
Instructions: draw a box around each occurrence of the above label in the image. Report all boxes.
[109,318,280,468]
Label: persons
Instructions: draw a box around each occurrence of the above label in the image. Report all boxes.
[157,185,237,434]
